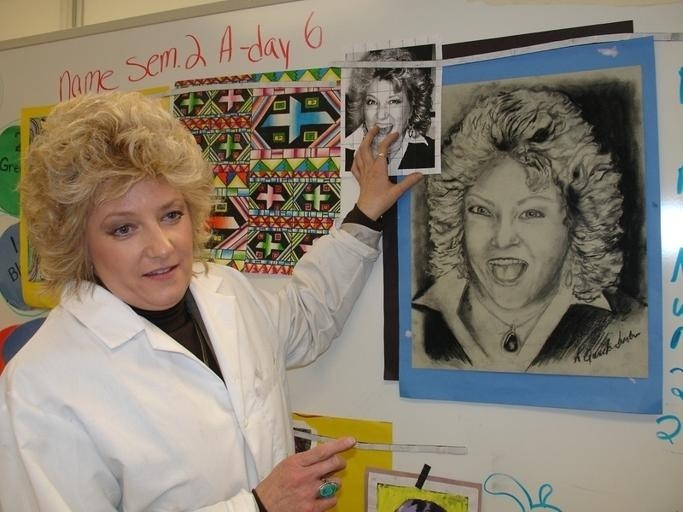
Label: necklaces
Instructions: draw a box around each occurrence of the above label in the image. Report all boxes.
[467,284,553,357]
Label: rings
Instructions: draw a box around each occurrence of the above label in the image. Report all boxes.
[316,477,341,503]
[374,151,390,160]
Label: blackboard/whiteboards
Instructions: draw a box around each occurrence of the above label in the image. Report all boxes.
[0,0,683,512]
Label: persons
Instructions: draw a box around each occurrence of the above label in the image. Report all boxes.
[411,86,648,377]
[1,86,420,512]
[345,49,435,171]
